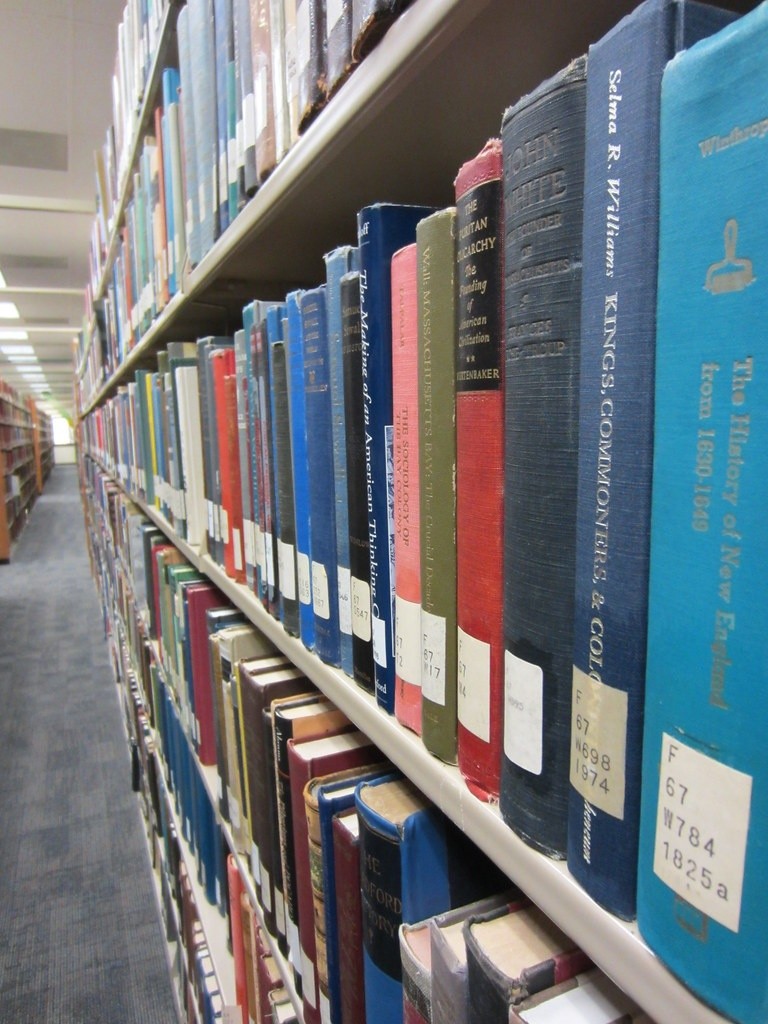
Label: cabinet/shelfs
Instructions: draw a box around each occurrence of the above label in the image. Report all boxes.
[0,0,768,1024]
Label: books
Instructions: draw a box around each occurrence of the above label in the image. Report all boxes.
[76,0,767,1024]
[0,379,55,545]
